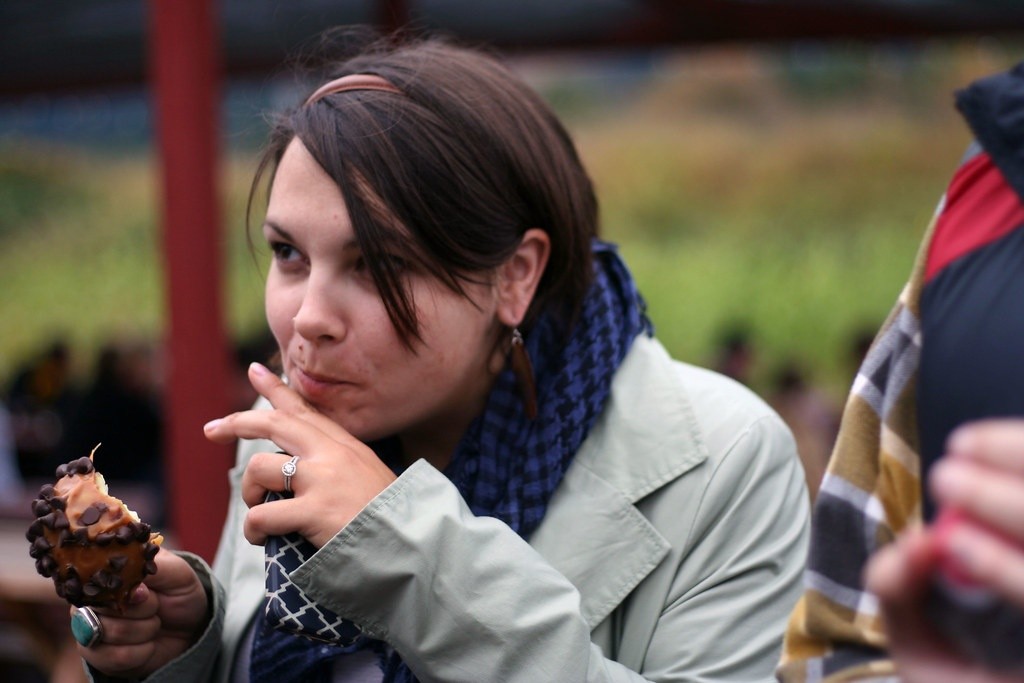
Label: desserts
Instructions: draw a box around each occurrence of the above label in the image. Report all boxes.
[26,444,163,614]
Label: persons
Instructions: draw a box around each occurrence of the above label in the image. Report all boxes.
[70,28,810,683]
[0,313,884,683]
[776,60,1024,683]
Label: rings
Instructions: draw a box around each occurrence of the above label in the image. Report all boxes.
[281,456,300,490]
[71,606,103,648]
